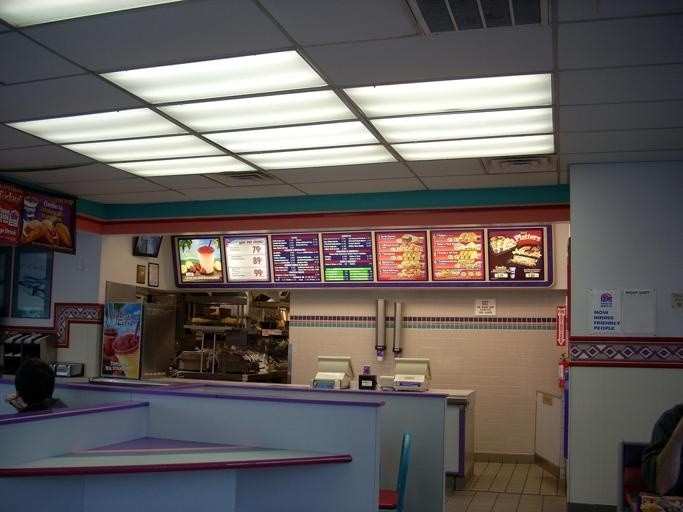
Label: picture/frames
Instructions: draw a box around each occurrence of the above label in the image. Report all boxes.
[0,243,52,320]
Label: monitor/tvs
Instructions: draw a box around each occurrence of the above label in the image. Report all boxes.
[315,356,354,389]
[133,236,163,258]
[393,358,431,392]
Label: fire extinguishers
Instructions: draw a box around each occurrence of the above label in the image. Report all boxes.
[558,353,569,388]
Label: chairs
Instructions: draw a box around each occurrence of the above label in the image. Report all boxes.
[377,433,412,512]
[621,442,651,511]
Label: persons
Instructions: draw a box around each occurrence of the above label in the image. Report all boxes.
[639,402,683,497]
[4,357,67,413]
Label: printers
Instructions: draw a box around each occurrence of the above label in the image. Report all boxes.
[49,361,84,377]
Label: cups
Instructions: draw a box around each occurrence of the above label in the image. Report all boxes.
[197,245,214,274]
[102,328,117,356]
[112,332,141,379]
[22,195,40,224]
